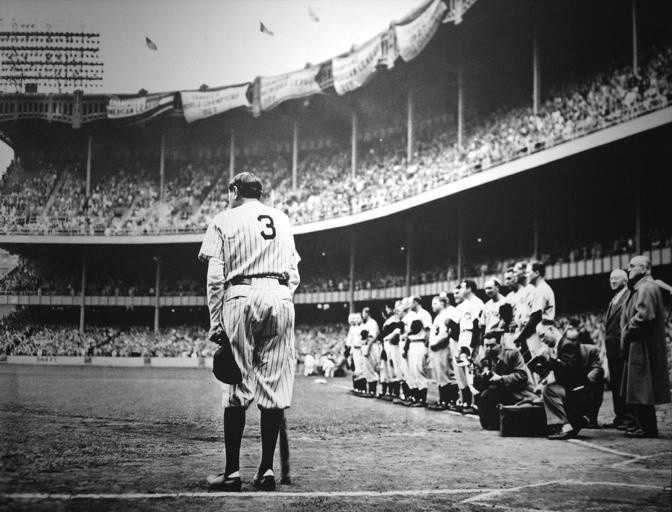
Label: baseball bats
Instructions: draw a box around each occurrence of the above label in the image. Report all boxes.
[279,417,290,485]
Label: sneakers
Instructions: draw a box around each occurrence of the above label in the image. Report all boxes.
[206,470,242,491]
[252,470,276,491]
[349,387,480,415]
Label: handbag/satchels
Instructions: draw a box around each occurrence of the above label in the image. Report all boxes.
[499,399,548,440]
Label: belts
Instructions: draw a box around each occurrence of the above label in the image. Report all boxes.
[225,276,291,286]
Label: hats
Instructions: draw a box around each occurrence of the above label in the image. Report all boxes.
[382,322,405,337]
[211,331,243,385]
[498,304,514,324]
[408,320,422,336]
[360,330,369,341]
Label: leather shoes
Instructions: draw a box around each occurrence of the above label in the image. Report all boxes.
[626,427,660,439]
[547,427,577,440]
[616,418,636,430]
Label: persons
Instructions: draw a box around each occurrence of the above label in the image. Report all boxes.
[0,35,670,235]
[1,238,669,358]
[341,263,604,441]
[618,255,668,438]
[194,171,302,492]
[600,266,626,432]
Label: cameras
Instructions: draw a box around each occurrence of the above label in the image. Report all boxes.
[528,356,552,383]
